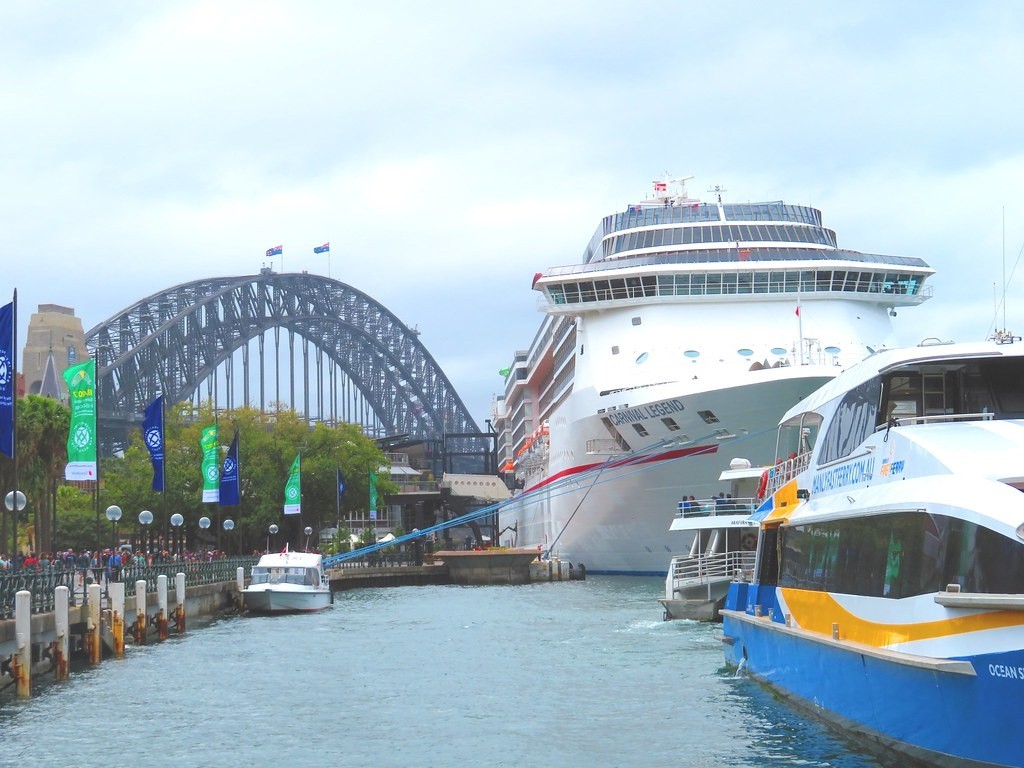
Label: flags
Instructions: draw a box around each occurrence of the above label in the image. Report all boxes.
[656,181,666,191]
[266,245,282,256]
[314,243,330,254]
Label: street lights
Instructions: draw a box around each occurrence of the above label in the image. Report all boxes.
[269,524,279,553]
[170,513,183,563]
[223,519,234,559]
[139,511,153,567]
[199,517,211,562]
[304,527,312,553]
[106,505,122,581]
[5,490,26,575]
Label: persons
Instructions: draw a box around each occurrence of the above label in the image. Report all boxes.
[0,545,322,582]
[679,493,735,519]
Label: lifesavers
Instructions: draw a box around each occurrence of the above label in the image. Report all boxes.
[741,533,758,550]
[756,471,768,497]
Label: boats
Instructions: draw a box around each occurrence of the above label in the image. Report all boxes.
[239,542,335,614]
[717,326,1024,768]
[657,457,774,622]
[489,170,936,577]
